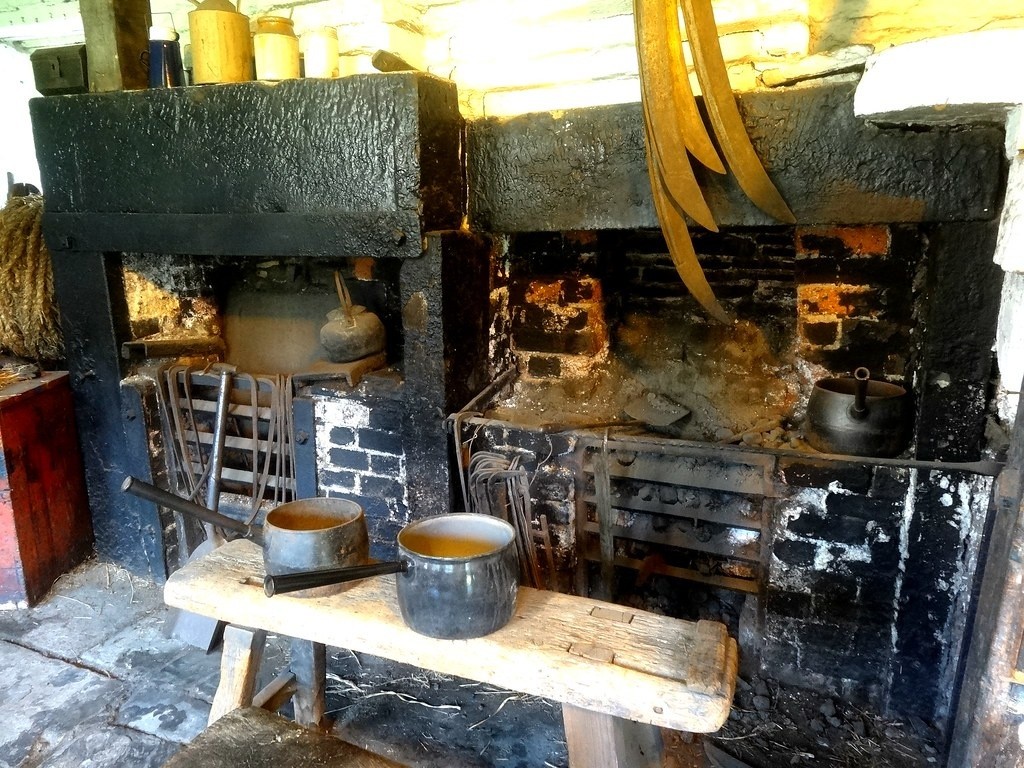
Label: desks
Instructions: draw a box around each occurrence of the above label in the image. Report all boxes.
[158,704,413,768]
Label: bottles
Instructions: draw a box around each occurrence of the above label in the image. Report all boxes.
[253,16,300,80]
[302,25,343,79]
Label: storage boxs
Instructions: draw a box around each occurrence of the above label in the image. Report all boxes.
[31,44,88,96]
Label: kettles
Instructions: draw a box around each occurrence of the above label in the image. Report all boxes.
[320,271,387,363]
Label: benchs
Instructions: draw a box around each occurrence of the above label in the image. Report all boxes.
[162,537,740,768]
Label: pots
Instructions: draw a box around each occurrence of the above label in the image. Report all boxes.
[805,367,906,457]
[121,476,369,598]
[263,513,519,639]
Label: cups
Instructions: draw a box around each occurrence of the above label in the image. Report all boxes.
[138,39,185,90]
[185,10,254,85]
